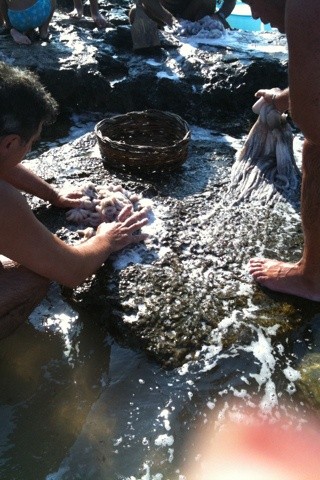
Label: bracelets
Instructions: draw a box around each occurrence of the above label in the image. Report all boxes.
[272,92,277,108]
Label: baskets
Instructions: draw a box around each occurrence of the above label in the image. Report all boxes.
[94,109,191,178]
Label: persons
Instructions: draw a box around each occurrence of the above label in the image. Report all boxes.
[0,0,320,339]
[0,62,155,339]
